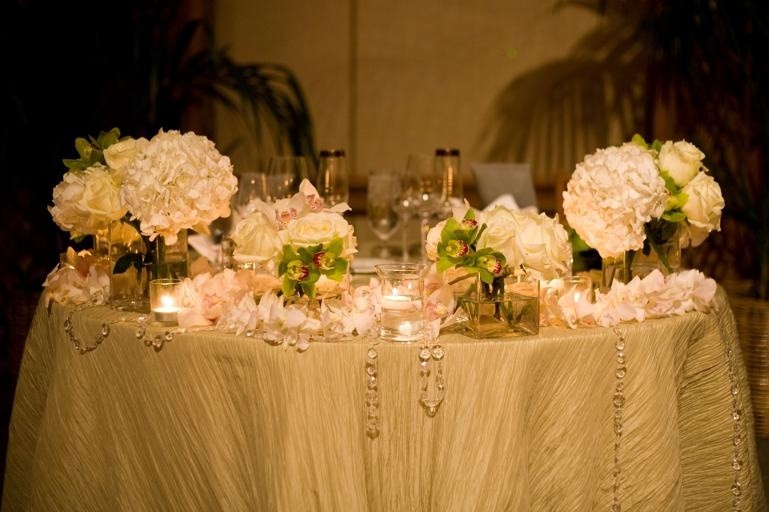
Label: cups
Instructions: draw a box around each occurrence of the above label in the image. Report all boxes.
[237,148,349,217]
[563,277,592,305]
[149,278,187,327]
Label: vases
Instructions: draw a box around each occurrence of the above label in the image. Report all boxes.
[93,228,189,314]
[600,238,681,293]
[254,256,350,320]
[444,271,540,339]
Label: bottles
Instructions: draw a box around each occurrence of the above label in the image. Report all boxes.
[373,263,432,342]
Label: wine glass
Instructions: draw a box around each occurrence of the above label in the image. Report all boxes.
[366,148,463,262]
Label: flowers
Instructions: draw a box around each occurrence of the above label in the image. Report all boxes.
[39,247,110,312]
[176,178,381,354]
[559,268,717,330]
[562,132,726,284]
[419,192,576,339]
[38,119,241,275]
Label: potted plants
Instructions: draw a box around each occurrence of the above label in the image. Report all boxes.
[631,1,767,440]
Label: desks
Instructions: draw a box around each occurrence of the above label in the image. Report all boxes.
[1,256,766,512]
